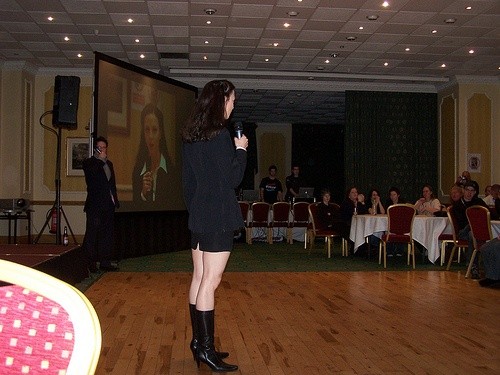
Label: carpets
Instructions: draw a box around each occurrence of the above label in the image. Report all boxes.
[117,242,467,272]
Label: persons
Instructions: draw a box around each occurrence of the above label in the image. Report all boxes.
[132,105,177,208]
[259,163,500,287]
[179,80,248,372]
[83,136,119,273]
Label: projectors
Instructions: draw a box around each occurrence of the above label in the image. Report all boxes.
[0,199,30,210]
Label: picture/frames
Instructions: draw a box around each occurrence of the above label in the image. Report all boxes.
[65,137,91,176]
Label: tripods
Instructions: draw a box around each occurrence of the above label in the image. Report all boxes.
[32,125,79,245]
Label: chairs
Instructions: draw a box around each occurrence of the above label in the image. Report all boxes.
[234,201,349,259]
[440,205,469,270]
[465,204,492,278]
[378,204,417,269]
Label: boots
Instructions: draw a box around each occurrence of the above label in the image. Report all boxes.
[188,302,230,360]
[194,307,239,372]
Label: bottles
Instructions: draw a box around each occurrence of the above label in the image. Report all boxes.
[372,200,377,215]
[63,226,69,246]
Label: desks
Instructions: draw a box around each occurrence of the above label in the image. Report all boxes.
[349,213,454,263]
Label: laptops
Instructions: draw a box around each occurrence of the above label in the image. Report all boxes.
[296,187,314,196]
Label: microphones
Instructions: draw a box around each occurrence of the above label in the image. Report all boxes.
[233,121,243,139]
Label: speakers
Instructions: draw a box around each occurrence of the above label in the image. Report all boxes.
[478,237,500,280]
[52,75,80,128]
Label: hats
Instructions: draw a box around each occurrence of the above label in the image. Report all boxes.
[464,181,477,190]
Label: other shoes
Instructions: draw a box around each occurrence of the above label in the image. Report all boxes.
[396,252,402,257]
[101,261,120,271]
[387,253,394,257]
[479,276,498,286]
[84,262,99,273]
